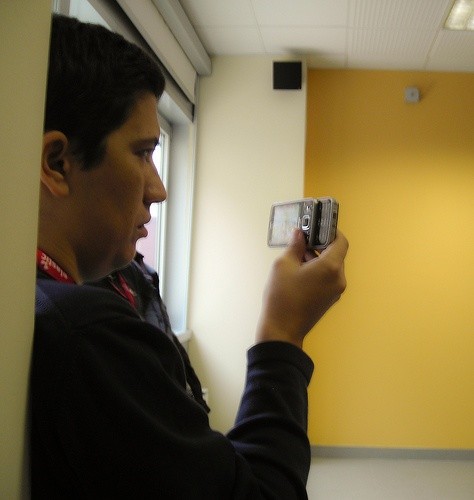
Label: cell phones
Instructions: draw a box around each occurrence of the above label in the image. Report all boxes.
[266,197,339,250]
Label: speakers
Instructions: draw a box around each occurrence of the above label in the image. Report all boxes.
[273,62,302,89]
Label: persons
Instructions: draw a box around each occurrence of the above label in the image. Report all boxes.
[102,252,211,414]
[30,14,350,500]
